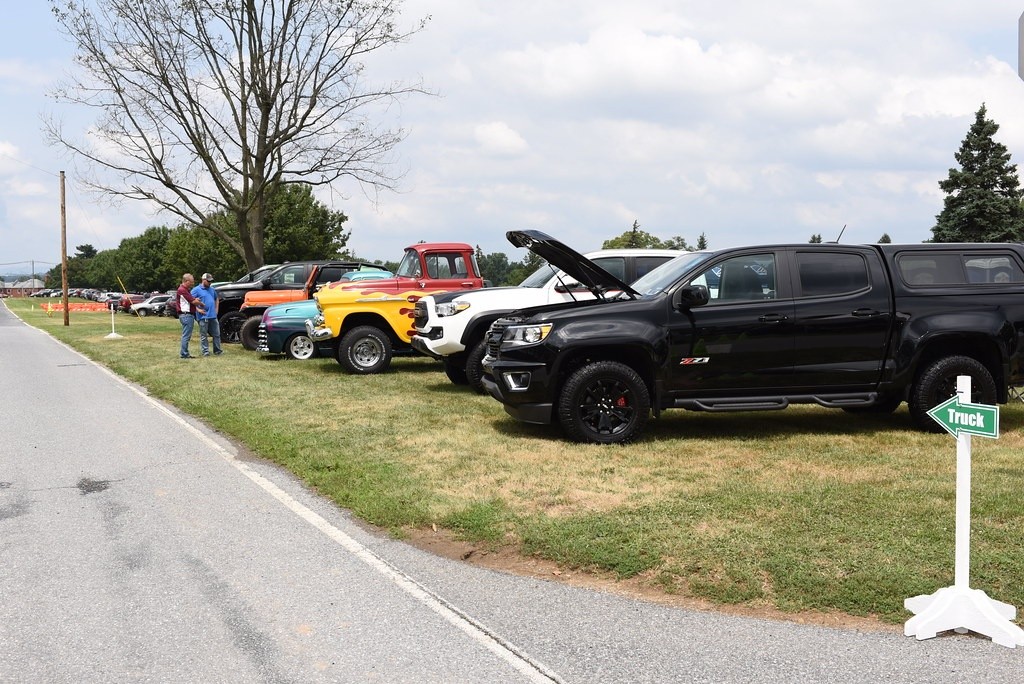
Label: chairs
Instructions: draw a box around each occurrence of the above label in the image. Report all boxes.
[737,266,763,300]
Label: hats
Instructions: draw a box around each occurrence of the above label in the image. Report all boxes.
[202,273,214,280]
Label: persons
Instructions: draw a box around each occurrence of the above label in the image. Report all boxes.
[176,273,206,359]
[191,273,223,357]
[76,291,81,297]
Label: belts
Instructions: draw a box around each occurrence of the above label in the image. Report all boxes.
[180,312,191,314]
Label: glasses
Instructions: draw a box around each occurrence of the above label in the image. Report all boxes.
[206,280,212,282]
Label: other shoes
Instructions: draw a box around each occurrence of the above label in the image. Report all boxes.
[214,349,224,356]
[203,351,210,356]
[180,354,196,358]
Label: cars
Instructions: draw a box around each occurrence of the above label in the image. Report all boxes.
[33,281,234,319]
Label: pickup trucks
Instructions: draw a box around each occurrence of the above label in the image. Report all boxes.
[306,243,494,374]
[258,271,408,362]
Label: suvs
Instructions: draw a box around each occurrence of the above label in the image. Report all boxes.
[413,248,691,392]
[238,264,357,352]
[483,231,1024,444]
